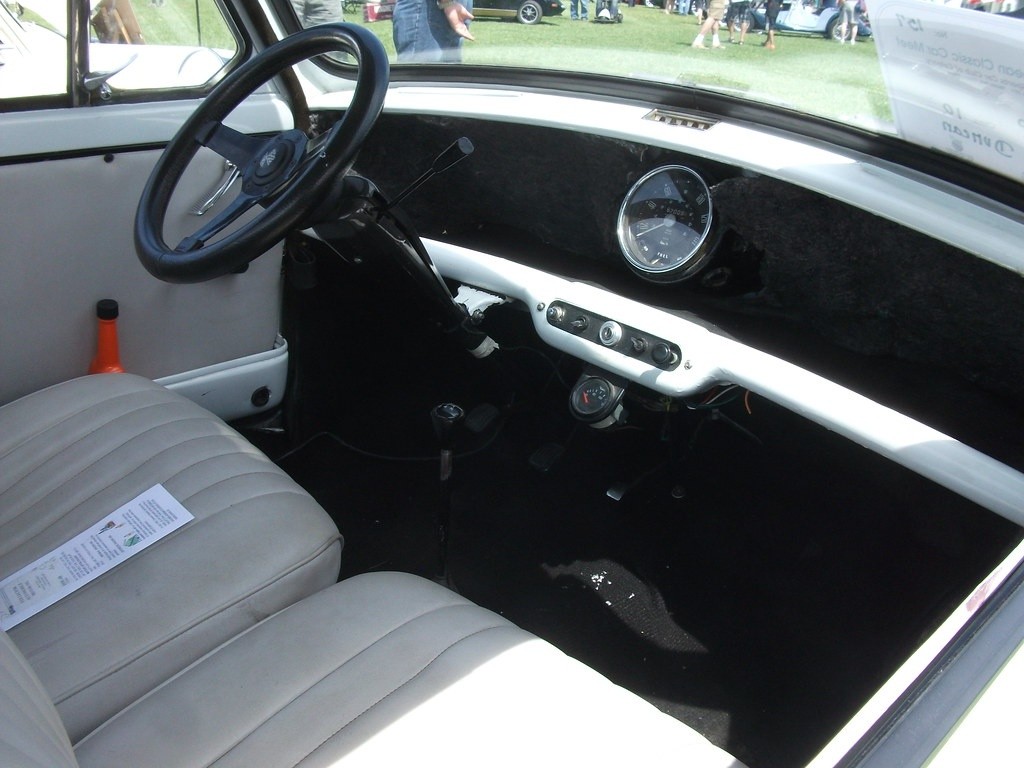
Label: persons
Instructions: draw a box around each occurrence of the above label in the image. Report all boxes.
[569,0,861,49]
[392,0,475,64]
[289,0,349,64]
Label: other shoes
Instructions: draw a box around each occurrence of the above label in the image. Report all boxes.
[691,42,708,49]
[761,41,775,49]
[712,43,726,49]
[729,37,737,45]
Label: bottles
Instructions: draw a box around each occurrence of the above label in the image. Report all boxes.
[88,298,127,374]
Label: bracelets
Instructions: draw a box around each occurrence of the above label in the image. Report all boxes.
[436,0,455,9]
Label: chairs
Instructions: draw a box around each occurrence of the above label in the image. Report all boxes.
[0,372,344,746]
[0,571,750,768]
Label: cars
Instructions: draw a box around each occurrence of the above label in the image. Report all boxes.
[473,0,566,25]
[733,0,871,41]
